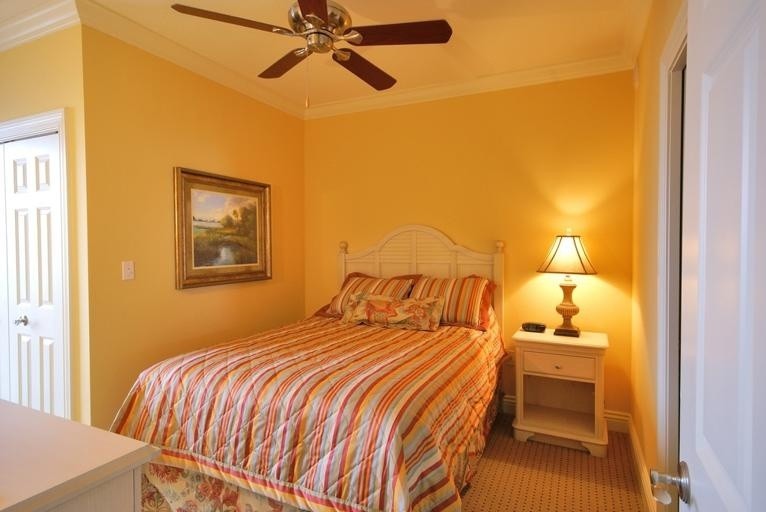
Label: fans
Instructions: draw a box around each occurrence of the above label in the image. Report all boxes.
[170,0,453,92]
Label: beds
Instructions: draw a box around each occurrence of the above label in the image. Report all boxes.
[139,222,506,512]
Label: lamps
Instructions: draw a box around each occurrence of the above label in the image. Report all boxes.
[536,236,598,337]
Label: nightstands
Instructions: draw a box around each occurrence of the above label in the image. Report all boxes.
[510,327,609,459]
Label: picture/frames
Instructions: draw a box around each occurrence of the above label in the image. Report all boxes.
[172,166,273,290]
